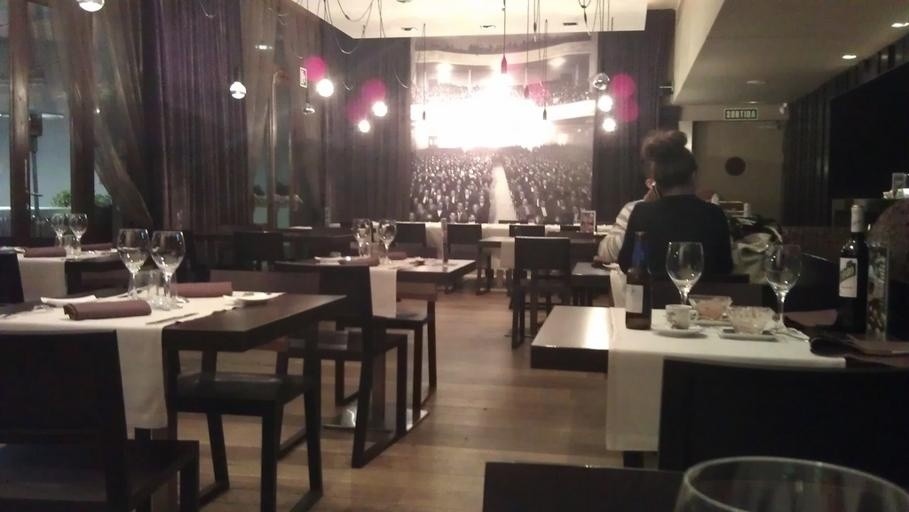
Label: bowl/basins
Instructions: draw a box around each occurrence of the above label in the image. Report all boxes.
[689,294,734,321]
[723,304,772,334]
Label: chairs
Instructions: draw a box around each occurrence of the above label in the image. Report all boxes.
[650,280,764,308]
[394,223,426,255]
[64,258,160,296]
[138,269,323,512]
[508,225,546,308]
[482,460,683,512]
[0,330,200,512]
[272,258,408,469]
[0,250,24,303]
[334,246,437,409]
[510,236,579,349]
[444,224,492,295]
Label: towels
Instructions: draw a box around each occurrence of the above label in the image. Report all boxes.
[16,252,67,303]
[116,295,245,447]
[62,298,152,321]
[24,246,67,260]
[388,250,410,262]
[425,222,442,250]
[543,230,565,237]
[371,258,400,322]
[606,327,666,453]
[491,235,516,284]
[169,280,233,297]
[348,254,381,268]
[82,242,115,252]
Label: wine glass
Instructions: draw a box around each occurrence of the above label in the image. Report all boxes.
[68,211,90,256]
[116,227,150,299]
[50,212,69,246]
[664,303,699,329]
[352,217,372,258]
[663,238,706,301]
[673,455,909,512]
[761,243,805,334]
[377,218,398,265]
[150,230,188,310]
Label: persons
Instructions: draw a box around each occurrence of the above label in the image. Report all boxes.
[409,53,596,222]
[617,129,733,281]
[598,175,652,263]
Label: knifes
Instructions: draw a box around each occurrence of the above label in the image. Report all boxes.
[146,312,198,326]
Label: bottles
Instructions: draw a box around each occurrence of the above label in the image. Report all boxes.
[623,227,654,331]
[835,202,872,339]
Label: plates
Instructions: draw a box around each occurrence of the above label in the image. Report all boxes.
[654,325,704,335]
[40,294,98,308]
[716,324,777,342]
[222,288,285,304]
